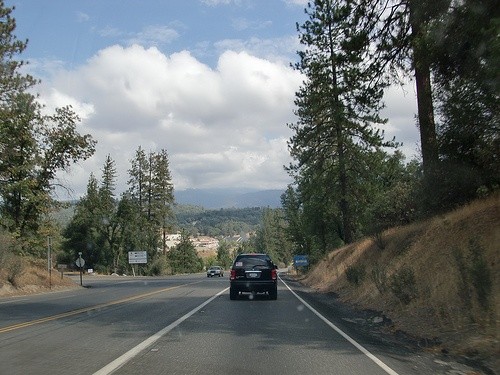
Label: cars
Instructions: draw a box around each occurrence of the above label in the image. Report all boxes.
[207,266,225,277]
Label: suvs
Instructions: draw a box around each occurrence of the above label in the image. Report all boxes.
[229,252,278,300]
